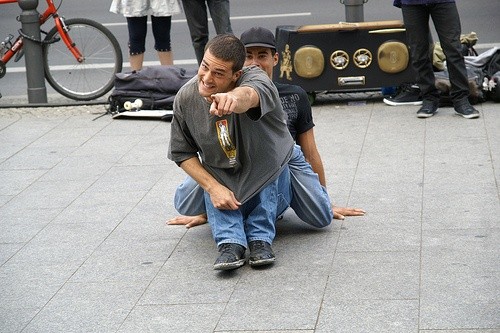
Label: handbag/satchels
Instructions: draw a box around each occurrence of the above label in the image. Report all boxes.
[107,64,198,110]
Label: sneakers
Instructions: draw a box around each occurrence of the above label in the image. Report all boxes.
[248,240,276,265]
[213,243,246,269]
[382,85,424,105]
[454,103,480,119]
[417,103,439,118]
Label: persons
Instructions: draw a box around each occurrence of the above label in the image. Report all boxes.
[181,0,234,68]
[392,0,480,119]
[167,26,367,270]
[109,0,184,73]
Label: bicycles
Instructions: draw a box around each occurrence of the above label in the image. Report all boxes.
[0,0,123,102]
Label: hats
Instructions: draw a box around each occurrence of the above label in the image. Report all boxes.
[240,27,277,53]
